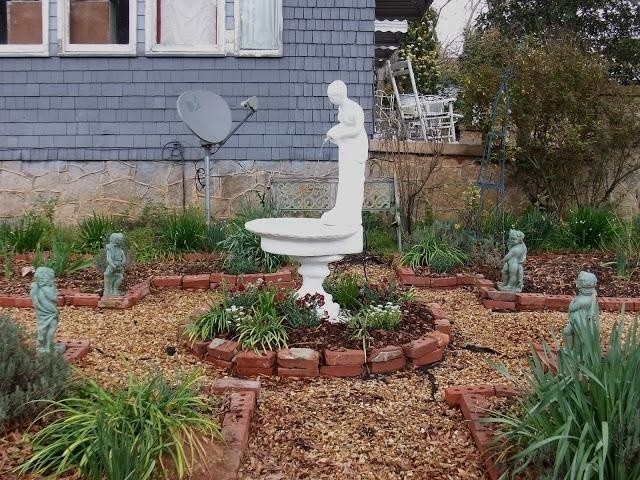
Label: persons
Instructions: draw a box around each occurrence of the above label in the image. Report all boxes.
[499,229,526,290]
[565,271,598,349]
[103,233,126,295]
[321,81,370,227]
[30,267,61,351]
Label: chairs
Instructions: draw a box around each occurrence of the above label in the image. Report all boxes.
[373,56,465,142]
